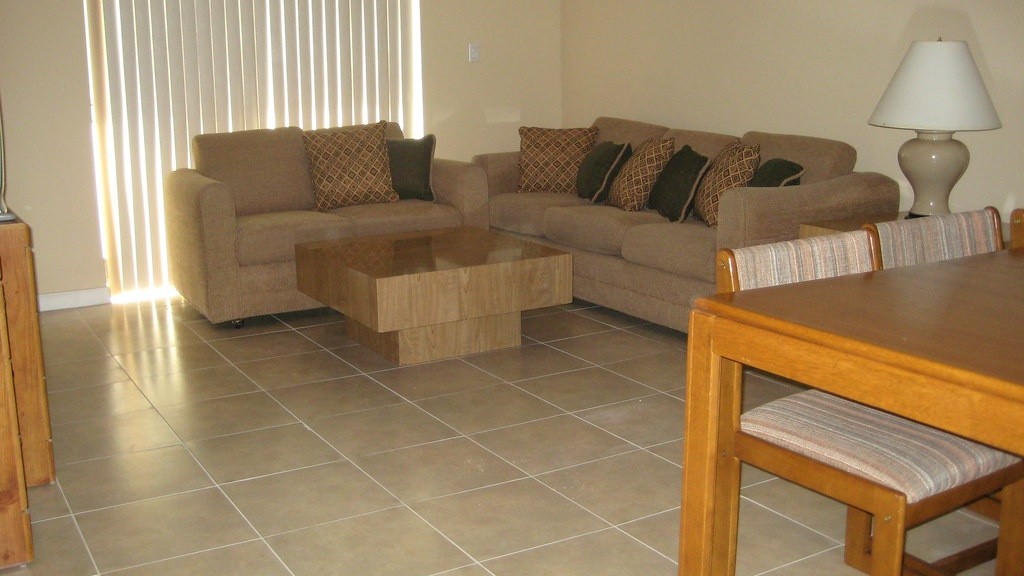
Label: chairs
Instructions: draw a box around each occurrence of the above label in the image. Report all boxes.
[715,228,1024,576]
[861,206,1004,270]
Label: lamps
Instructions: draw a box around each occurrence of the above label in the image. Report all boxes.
[867,37,1003,219]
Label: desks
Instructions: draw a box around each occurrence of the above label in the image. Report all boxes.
[677,249,1024,575]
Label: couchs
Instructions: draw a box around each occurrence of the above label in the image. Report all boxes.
[472,117,899,334]
[167,125,490,328]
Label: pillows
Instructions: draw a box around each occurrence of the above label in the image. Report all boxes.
[386,133,437,202]
[576,140,632,204]
[644,144,712,224]
[516,126,600,193]
[302,119,400,211]
[748,158,807,187]
[606,137,676,211]
[694,137,761,230]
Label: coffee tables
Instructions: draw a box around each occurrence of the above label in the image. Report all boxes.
[295,225,573,367]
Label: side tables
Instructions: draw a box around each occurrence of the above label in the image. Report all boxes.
[799,212,907,240]
